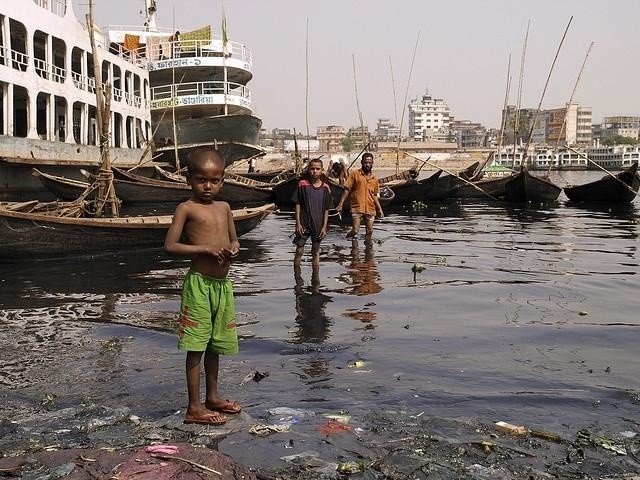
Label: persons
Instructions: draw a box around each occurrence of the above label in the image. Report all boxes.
[335,153,384,235]
[291,159,332,265]
[248,158,256,173]
[164,148,241,425]
[169,31,180,59]
[339,158,346,185]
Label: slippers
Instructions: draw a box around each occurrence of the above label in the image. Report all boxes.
[209,398,241,414]
[184,411,227,426]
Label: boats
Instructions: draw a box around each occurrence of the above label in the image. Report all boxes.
[561,161,640,205]
[27,0,594,225]
[0,1,275,263]
[99,0,265,175]
